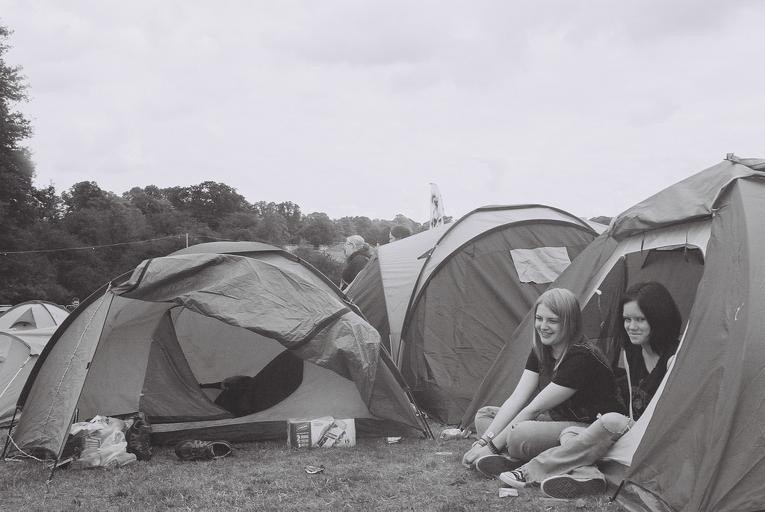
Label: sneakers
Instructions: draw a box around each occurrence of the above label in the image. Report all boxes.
[175,440,233,460]
[541,475,607,502]
[125,420,151,461]
[476,454,534,489]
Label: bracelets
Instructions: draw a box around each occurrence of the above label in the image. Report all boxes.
[477,429,501,455]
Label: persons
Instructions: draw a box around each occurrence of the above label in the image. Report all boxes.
[384,223,413,244]
[335,235,371,289]
[458,286,621,479]
[495,280,680,501]
[73,297,81,308]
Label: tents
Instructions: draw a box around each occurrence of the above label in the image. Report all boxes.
[343,201,613,425]
[0,299,71,427]
[448,152,765,512]
[4,242,438,494]
